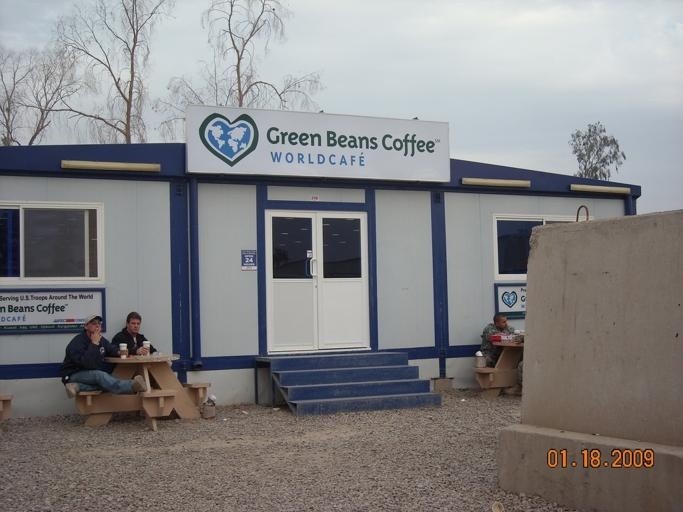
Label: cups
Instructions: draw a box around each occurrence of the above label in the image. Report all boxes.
[143,340,150,357]
[119,343,128,359]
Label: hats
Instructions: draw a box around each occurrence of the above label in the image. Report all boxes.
[84,312,103,326]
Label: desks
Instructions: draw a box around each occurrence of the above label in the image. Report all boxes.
[481,337,525,401]
[83,355,200,431]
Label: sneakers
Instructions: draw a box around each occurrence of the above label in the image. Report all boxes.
[131,374,147,393]
[65,382,80,399]
[502,382,523,396]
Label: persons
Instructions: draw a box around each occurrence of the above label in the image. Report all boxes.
[61,314,148,401]
[503,332,524,398]
[479,310,515,368]
[111,310,158,390]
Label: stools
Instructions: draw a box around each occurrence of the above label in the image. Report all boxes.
[137,388,178,419]
[185,380,211,406]
[0,395,15,423]
[76,388,102,417]
[470,361,498,391]
